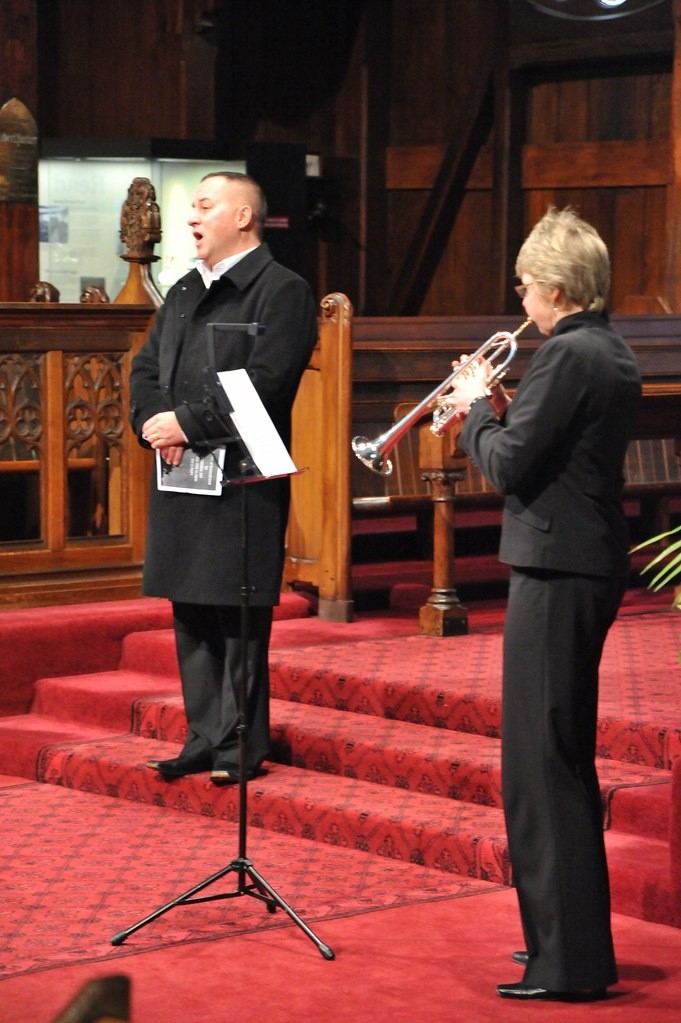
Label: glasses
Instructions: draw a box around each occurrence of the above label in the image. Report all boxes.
[514,281,534,298]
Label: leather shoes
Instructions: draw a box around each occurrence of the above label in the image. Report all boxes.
[496,974,606,1002]
[145,755,199,775]
[513,951,530,964]
[209,760,258,783]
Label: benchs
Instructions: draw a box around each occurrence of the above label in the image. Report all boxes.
[352,341,681,512]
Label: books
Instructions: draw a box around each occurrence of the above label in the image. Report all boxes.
[156,444,226,495]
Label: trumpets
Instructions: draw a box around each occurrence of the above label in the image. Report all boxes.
[351,318,533,476]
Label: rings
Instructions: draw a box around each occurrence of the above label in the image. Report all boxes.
[152,434,157,440]
[143,434,147,439]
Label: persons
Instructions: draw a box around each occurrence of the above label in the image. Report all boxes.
[128,172,319,783]
[446,204,642,1000]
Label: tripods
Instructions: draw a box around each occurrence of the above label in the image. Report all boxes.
[109,368,334,962]
